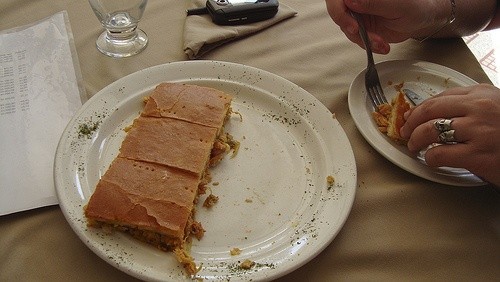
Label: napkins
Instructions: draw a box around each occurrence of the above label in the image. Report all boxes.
[181,0,299,60]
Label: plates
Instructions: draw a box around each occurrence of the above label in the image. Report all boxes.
[347,59,488,187]
[52,59,358,282]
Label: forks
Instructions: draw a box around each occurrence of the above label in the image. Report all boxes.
[350,10,390,110]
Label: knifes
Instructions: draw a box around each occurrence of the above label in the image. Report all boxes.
[403,88,424,106]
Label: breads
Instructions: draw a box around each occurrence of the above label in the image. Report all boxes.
[80,82,233,252]
[372,90,415,140]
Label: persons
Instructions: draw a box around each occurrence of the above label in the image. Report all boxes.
[325,0,500,186]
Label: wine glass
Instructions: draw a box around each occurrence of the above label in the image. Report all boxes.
[88,0,149,58]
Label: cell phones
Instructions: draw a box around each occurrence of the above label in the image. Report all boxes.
[186,0,279,26]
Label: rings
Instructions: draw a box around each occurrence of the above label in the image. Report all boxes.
[434,118,456,145]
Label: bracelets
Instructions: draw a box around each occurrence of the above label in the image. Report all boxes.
[418,0,458,44]
